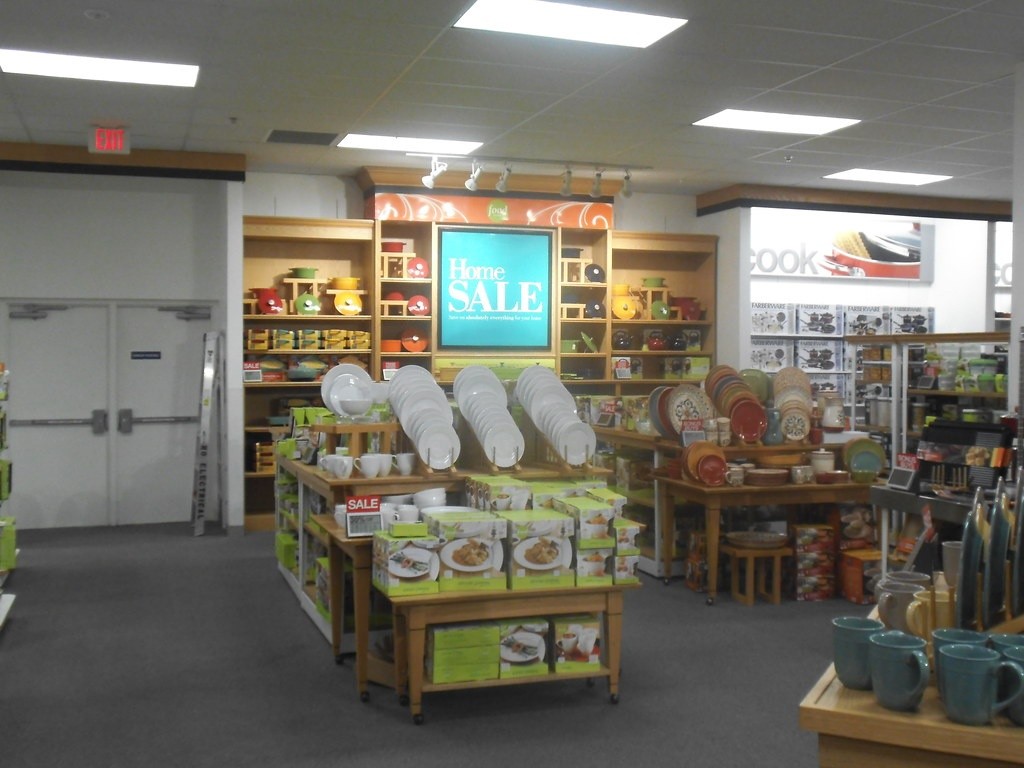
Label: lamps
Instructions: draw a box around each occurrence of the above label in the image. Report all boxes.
[560,171,572,196]
[589,173,602,198]
[422,163,445,189]
[465,164,483,192]
[620,176,632,198]
[495,169,511,193]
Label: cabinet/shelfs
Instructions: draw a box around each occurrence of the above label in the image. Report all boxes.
[241,212,718,533]
[752,318,1024,551]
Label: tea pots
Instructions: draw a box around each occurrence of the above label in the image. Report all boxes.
[612,329,689,351]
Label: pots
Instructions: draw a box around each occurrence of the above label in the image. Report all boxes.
[381,285,405,300]
[381,241,407,253]
[561,248,604,353]
[248,267,360,299]
[612,276,697,307]
[380,339,403,352]
[817,220,921,279]
[773,311,927,392]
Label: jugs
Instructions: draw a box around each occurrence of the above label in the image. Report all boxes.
[817,388,845,432]
[762,407,784,445]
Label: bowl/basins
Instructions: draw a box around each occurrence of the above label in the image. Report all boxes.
[580,519,608,574]
[384,487,446,509]
[853,470,875,482]
[339,399,373,417]
[815,469,849,484]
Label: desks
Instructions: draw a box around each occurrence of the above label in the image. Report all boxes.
[651,469,887,605]
[387,574,640,723]
[586,421,846,577]
[800,589,1024,768]
[274,445,611,666]
[313,507,648,702]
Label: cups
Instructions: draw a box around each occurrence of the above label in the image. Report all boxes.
[555,625,598,655]
[792,447,835,484]
[725,467,744,487]
[491,492,511,510]
[319,453,415,479]
[831,540,1024,727]
[332,503,418,531]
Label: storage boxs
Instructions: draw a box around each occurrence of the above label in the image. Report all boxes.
[274,396,648,685]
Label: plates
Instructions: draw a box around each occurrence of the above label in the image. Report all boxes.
[320,364,813,485]
[499,632,541,661]
[386,538,562,577]
[840,436,887,476]
